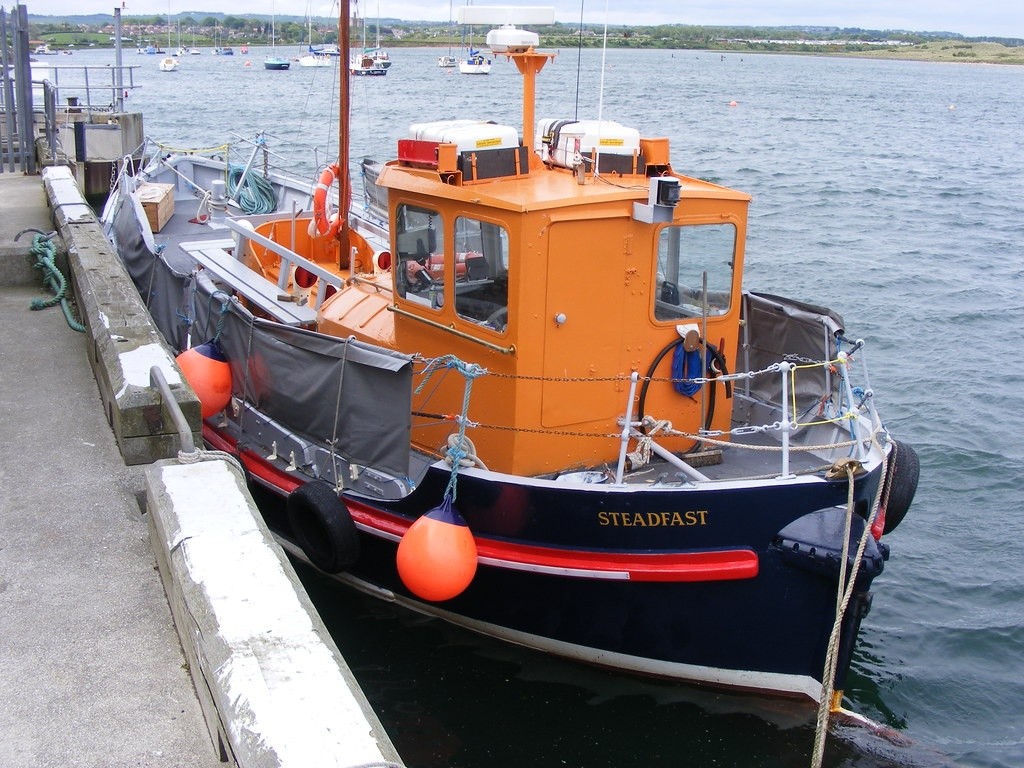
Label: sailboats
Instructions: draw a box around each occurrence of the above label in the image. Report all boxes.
[33,0,497,79]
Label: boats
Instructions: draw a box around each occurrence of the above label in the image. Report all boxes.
[99,0,921,747]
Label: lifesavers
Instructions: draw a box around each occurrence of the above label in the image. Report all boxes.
[283,479,358,575]
[884,439,920,536]
[315,165,352,240]
[405,252,484,282]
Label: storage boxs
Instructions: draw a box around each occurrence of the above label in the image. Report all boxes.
[133,181,175,233]
[411,119,520,156]
[535,116,640,170]
